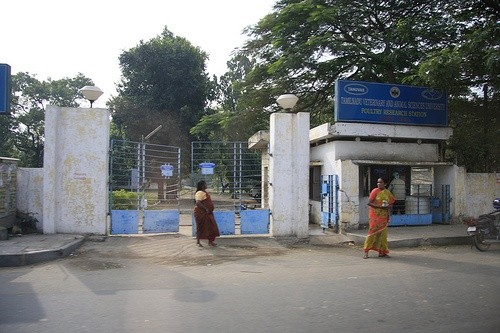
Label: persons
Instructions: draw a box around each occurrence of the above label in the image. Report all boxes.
[387,171,406,215]
[193,180,220,247]
[363,174,395,259]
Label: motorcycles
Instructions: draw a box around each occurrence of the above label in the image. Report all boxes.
[462,198,500,252]
